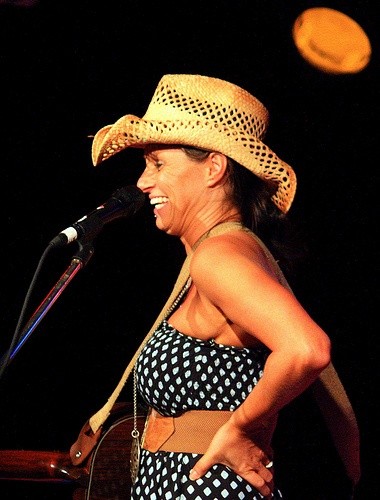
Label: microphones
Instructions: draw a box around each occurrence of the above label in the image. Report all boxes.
[49,185,146,248]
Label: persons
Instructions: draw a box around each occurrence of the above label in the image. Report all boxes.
[91,75,331,500]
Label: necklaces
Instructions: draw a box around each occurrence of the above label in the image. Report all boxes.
[128,276,193,484]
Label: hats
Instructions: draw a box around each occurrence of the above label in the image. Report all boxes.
[92,75,297,214]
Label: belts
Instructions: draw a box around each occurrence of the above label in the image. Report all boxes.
[139,406,274,454]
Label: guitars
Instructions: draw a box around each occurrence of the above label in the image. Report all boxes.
[0,390,355,500]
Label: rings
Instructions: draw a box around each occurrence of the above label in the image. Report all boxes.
[265,460,274,469]
